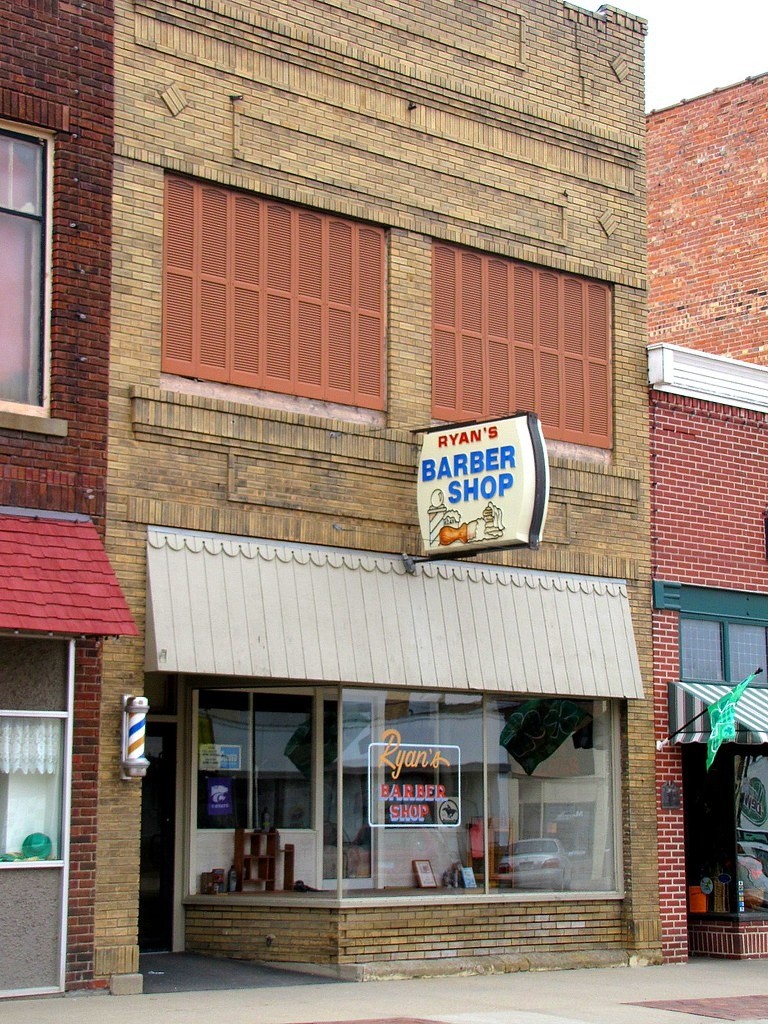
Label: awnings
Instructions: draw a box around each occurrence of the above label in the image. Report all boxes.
[666,682,768,747]
[140,529,646,701]
[0,513,142,638]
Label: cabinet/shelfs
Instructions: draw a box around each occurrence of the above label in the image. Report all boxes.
[466,816,514,889]
[234,827,295,891]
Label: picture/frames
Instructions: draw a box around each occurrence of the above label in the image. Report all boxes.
[412,860,437,888]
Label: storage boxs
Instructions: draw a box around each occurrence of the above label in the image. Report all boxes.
[688,886,708,912]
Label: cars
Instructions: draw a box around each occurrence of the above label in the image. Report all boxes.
[498,838,572,893]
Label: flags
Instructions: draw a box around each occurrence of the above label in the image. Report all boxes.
[285,707,369,777]
[705,674,756,773]
[497,695,592,777]
